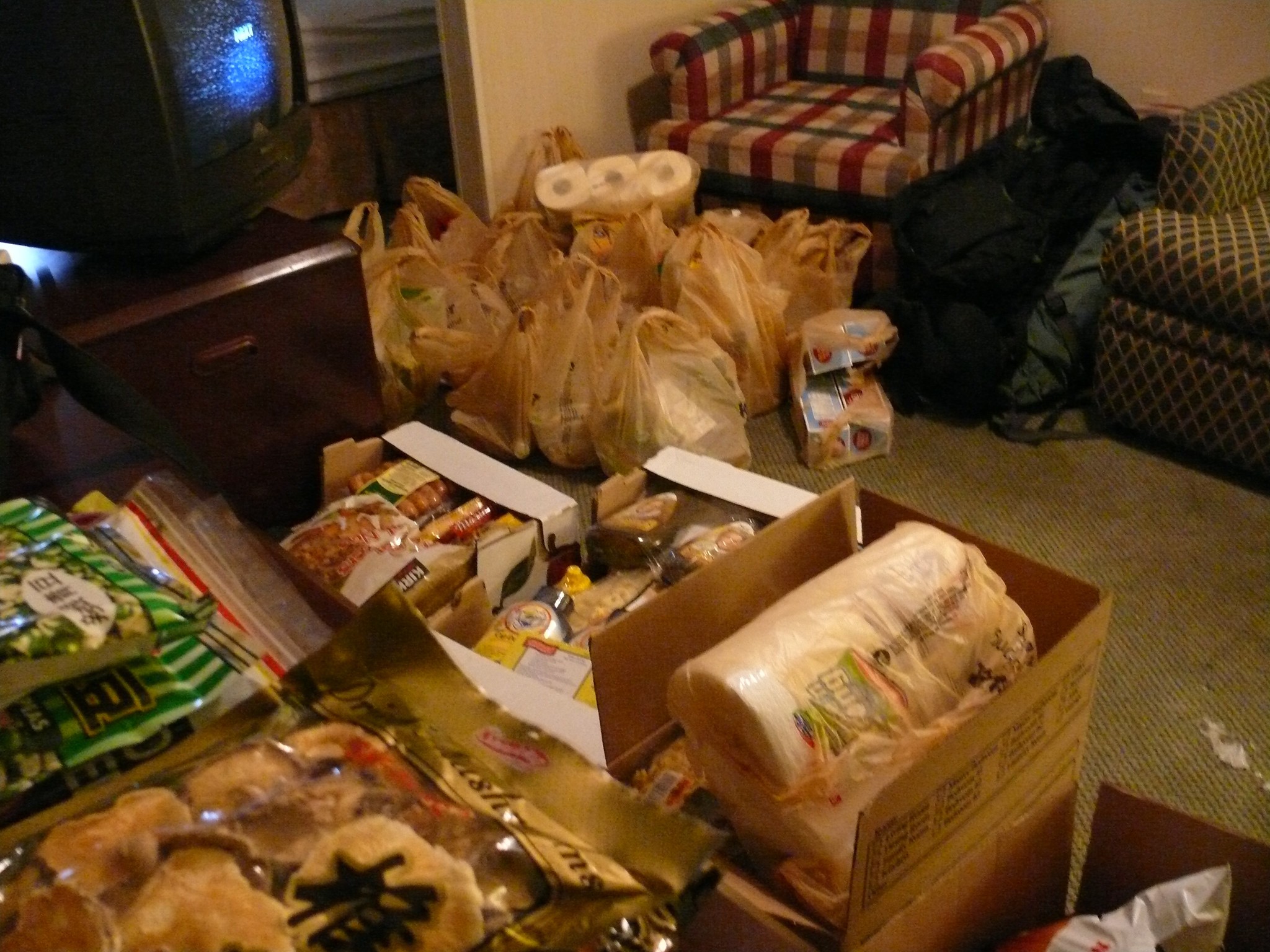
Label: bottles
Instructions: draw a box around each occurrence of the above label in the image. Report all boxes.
[472,566,591,671]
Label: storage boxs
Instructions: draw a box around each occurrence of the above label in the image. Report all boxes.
[843,317,888,363]
[833,368,889,460]
[858,779,1270,952]
[320,418,580,620]
[426,444,864,771]
[800,324,854,377]
[791,370,850,467]
[589,476,1116,952]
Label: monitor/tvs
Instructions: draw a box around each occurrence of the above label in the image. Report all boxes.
[0,0,312,268]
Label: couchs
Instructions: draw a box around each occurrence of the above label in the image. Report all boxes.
[639,0,1050,416]
[1088,75,1270,484]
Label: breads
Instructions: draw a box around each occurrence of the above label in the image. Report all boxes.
[590,491,758,588]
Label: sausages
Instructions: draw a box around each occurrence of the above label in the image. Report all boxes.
[346,457,448,518]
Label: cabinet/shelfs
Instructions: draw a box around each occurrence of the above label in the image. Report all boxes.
[30,205,382,531]
[266,74,461,222]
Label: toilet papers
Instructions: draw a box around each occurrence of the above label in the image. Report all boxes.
[683,602,1039,894]
[533,161,588,229]
[638,149,693,215]
[667,523,1001,798]
[587,155,638,204]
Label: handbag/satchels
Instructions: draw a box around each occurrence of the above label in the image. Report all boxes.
[991,172,1158,444]
[343,128,869,476]
